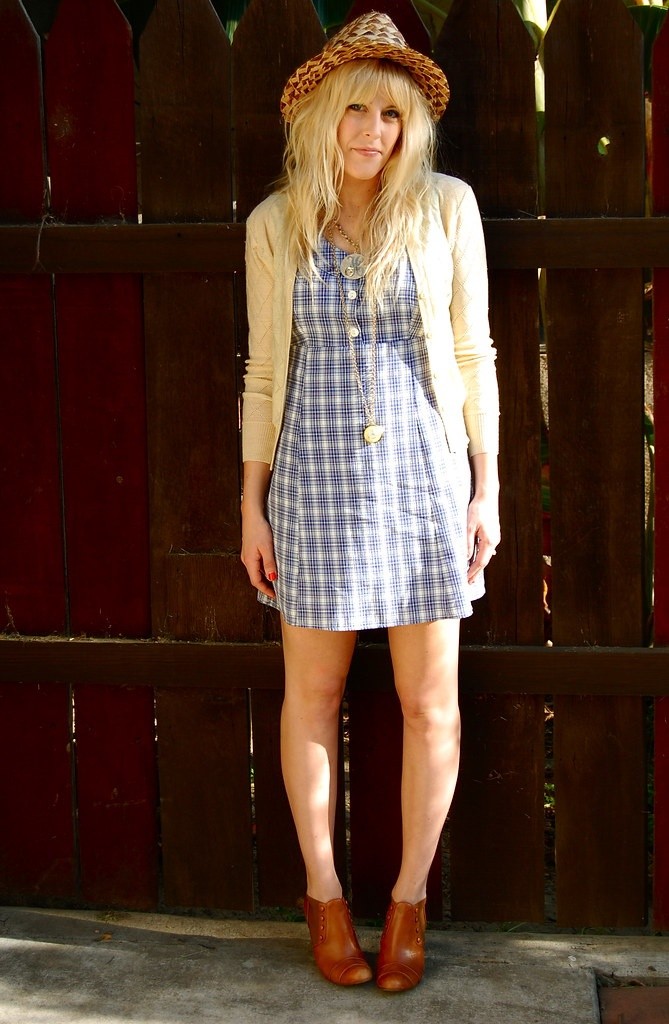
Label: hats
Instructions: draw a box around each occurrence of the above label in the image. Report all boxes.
[280,11,450,132]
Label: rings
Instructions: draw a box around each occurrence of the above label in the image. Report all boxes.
[492,549,497,556]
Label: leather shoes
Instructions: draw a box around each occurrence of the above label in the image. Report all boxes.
[303,891,372,985]
[375,895,426,992]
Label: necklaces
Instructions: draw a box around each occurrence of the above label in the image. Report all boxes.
[329,201,389,446]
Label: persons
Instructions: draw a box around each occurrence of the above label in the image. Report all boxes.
[240,5,503,994]
